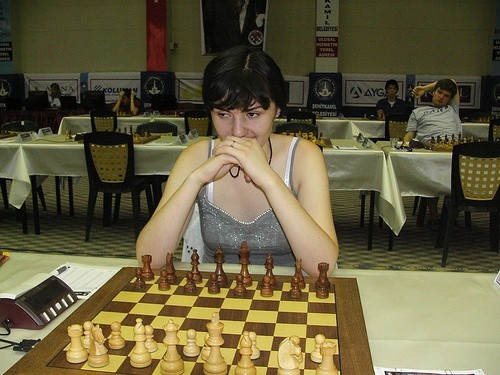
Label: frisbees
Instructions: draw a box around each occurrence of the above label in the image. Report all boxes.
[3,266,375,375]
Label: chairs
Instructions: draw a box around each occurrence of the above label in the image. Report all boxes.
[0,121,46,214]
[385,112,500,267]
[271,111,318,141]
[83,109,213,242]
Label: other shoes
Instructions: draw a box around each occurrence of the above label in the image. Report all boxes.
[429,217,441,231]
[452,219,459,233]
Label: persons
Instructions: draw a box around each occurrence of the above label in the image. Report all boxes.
[376,80,408,117]
[404,78,462,229]
[113,89,141,115]
[136,45,339,278]
[47,83,64,108]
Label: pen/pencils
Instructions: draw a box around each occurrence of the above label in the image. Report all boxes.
[336,146,340,149]
[52,266,67,276]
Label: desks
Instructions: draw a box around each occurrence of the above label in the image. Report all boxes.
[276,117,388,138]
[461,122,490,141]
[0,250,499,375]
[324,139,394,250]
[377,141,452,251]
[0,136,189,234]
[57,114,183,137]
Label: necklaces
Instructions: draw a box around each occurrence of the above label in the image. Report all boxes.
[229,137,273,178]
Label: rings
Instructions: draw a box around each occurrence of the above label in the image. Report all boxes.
[231,141,236,147]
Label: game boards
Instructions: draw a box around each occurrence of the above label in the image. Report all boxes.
[294,137,333,149]
[425,138,479,151]
[115,113,134,117]
[133,135,161,144]
